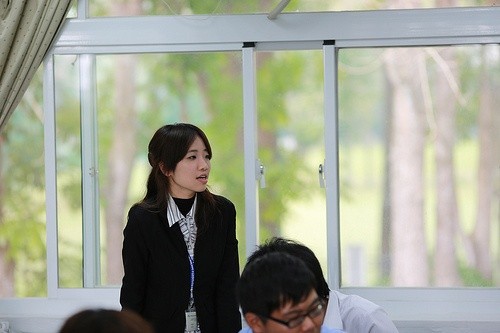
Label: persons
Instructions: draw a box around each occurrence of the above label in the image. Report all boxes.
[237,237,399,333]
[121,123,242,333]
[57,309,153,333]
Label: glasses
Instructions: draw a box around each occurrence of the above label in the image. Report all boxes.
[254,299,324,329]
